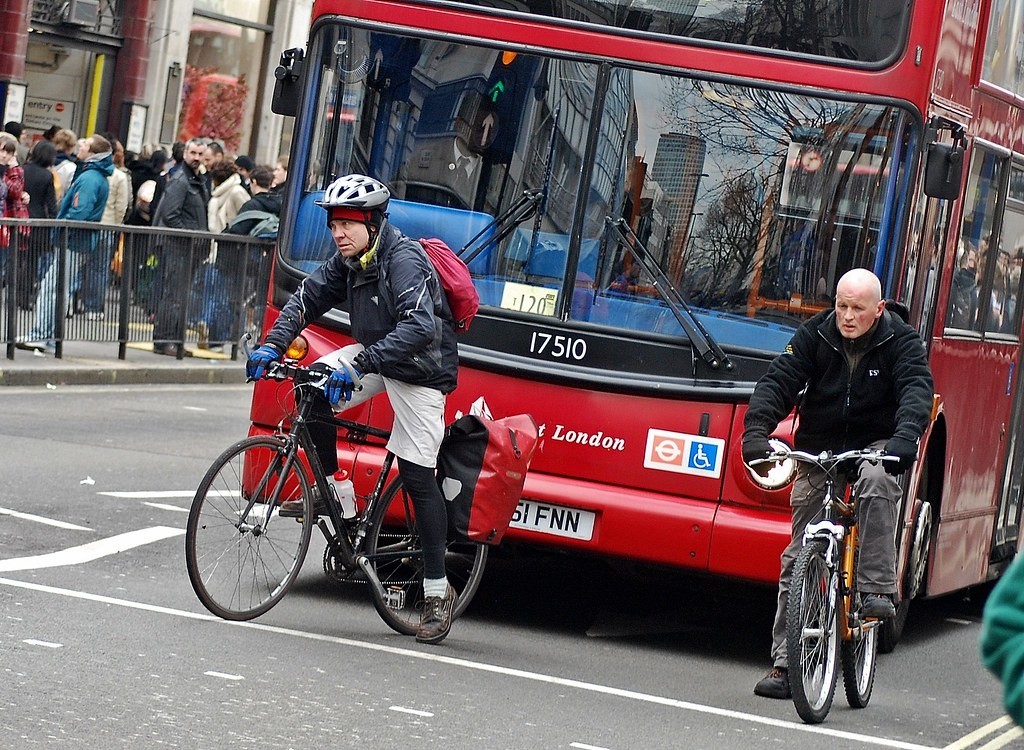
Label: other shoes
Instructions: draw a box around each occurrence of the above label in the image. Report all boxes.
[86,312,103,322]
[154,341,193,357]
[16,336,47,352]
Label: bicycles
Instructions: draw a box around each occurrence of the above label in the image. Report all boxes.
[185,332,487,637]
[748,448,888,726]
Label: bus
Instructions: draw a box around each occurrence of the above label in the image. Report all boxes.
[241,0,1024,653]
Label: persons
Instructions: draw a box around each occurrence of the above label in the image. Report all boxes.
[202,142,247,196]
[233,155,256,195]
[196,166,283,350]
[37,124,86,317]
[0,131,31,295]
[81,132,128,320]
[17,141,58,310]
[203,158,252,337]
[743,268,935,700]
[14,134,115,351]
[5,121,30,170]
[385,92,505,211]
[110,140,169,323]
[603,247,645,297]
[164,138,210,357]
[977,546,1024,730]
[247,173,458,645]
[170,141,185,166]
[905,230,1024,339]
[269,155,290,200]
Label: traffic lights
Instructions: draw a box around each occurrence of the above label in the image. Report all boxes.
[469,50,539,165]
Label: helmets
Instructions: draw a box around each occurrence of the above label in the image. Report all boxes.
[314,173,390,213]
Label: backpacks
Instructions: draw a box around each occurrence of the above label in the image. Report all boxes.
[394,237,479,332]
[229,209,280,249]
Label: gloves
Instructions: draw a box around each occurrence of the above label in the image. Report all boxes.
[324,361,365,404]
[742,429,776,476]
[245,343,283,381]
[881,431,920,477]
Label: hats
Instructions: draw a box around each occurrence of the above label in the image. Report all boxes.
[326,205,375,230]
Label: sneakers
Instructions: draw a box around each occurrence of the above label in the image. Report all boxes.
[415,583,459,645]
[860,592,897,619]
[279,485,339,518]
[754,668,789,699]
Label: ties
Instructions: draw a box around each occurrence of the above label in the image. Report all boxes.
[453,157,471,194]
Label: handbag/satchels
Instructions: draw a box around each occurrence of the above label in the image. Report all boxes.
[435,412,539,546]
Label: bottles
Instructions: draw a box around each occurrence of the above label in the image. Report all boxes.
[333,468,358,519]
